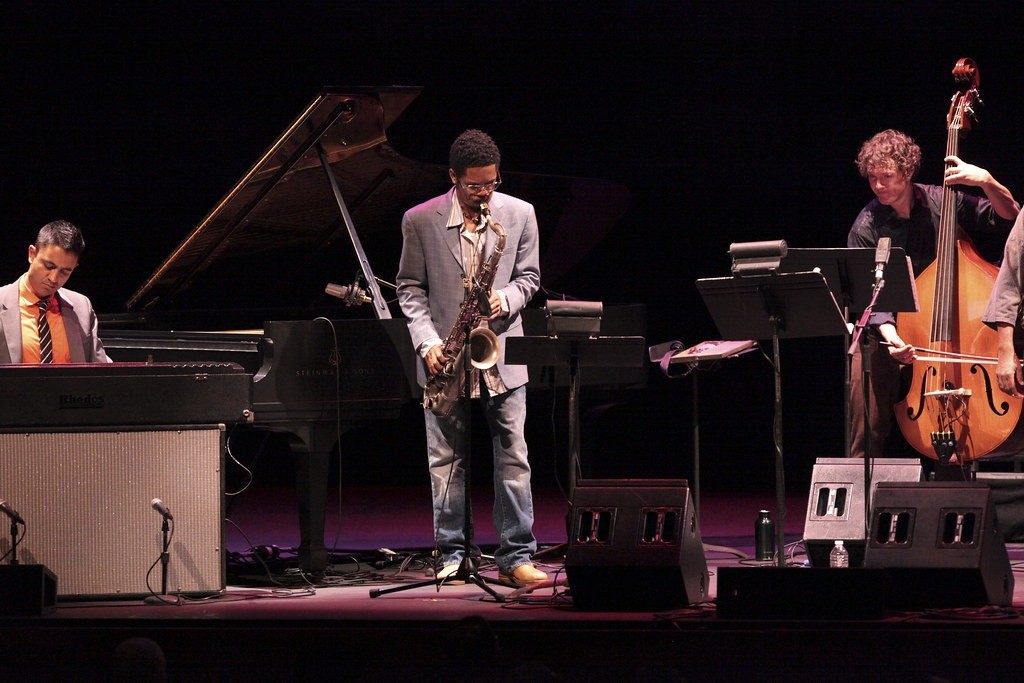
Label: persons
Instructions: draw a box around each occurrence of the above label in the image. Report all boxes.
[981,205,1023,398]
[0,221,112,363]
[846,128,1021,458]
[394,130,547,585]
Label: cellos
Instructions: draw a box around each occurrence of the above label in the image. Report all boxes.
[894,57,1024,464]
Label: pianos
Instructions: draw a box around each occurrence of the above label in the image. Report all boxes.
[0,362,254,423]
[96,85,424,575]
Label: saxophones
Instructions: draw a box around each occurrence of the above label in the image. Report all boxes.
[420,202,508,419]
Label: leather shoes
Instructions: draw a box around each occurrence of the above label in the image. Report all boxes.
[498,564,548,585]
[436,564,466,585]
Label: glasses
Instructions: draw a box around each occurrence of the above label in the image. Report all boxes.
[455,170,502,192]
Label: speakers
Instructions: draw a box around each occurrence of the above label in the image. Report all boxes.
[803,457,1024,610]
[566,479,710,608]
[0,422,227,601]
[0,564,57,614]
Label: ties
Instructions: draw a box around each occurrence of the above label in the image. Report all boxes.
[35,300,54,364]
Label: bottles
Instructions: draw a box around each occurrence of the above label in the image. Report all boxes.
[755,509,775,561]
[830,541,849,568]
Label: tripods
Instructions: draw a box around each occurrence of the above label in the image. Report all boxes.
[370,289,533,603]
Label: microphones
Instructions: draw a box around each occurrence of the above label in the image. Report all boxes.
[325,282,373,303]
[875,237,891,280]
[472,276,492,316]
[0,499,24,525]
[151,498,174,520]
